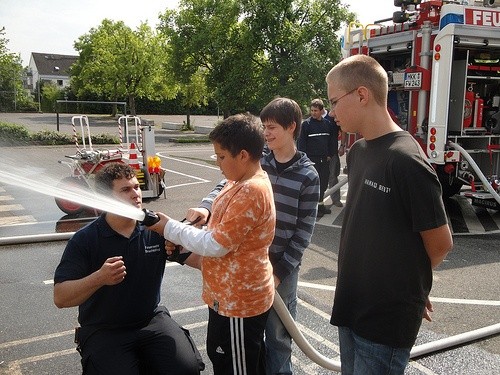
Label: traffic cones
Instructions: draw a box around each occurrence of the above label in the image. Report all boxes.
[128,143,141,171]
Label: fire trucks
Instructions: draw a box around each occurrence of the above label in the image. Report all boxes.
[337,1,500,214]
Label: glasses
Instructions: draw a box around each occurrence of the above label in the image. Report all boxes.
[329,88,359,109]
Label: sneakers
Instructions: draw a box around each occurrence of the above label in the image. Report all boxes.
[317,205,331,217]
[333,201,343,207]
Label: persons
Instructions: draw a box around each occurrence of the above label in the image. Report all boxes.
[325,54,454,375]
[149,113,277,375]
[298,99,343,217]
[52,161,206,375]
[185,98,320,375]
